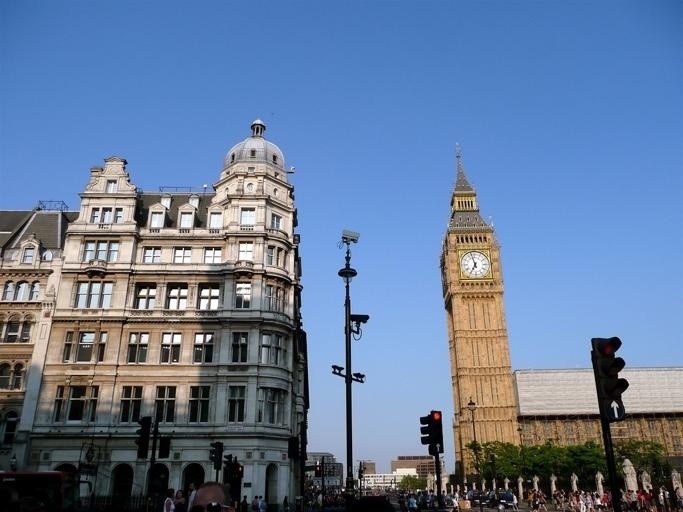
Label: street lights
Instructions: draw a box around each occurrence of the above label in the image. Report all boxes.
[332,231,369,494]
[467,397,478,465]
[518,428,527,492]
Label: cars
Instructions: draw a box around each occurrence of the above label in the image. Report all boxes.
[466,490,513,509]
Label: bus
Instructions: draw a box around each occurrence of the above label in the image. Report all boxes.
[1,469,65,512]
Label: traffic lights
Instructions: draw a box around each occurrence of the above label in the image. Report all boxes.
[210,441,223,472]
[591,336,628,421]
[359,470,363,478]
[315,465,321,477]
[135,416,151,460]
[225,455,243,501]
[420,411,444,457]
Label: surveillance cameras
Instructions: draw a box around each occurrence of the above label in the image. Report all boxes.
[341,229,360,243]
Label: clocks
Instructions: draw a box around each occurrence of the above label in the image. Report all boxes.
[456,247,494,282]
